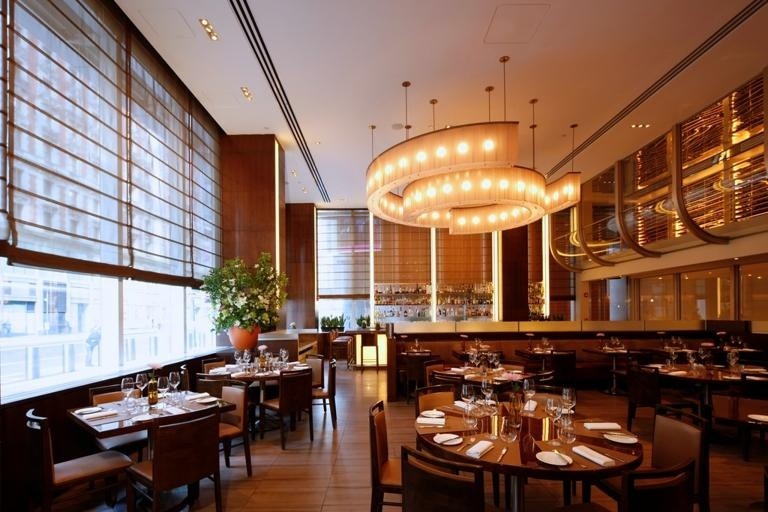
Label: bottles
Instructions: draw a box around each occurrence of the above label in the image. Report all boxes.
[260,353,267,370]
[148,378,158,404]
[375,283,431,317]
[436,283,493,320]
[510,394,521,432]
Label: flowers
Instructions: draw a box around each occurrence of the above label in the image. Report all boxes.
[200,252,289,330]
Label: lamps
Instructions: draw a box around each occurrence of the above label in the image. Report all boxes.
[366,54,582,228]
[403,94,547,236]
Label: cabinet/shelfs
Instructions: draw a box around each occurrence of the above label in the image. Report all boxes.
[528,281,544,320]
[435,280,494,324]
[372,284,433,321]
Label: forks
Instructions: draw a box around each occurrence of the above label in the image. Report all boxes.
[456,437,476,451]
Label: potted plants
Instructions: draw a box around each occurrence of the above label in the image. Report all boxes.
[320,313,347,332]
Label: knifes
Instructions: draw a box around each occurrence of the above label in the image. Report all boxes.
[553,448,569,464]
[602,432,638,438]
[587,444,626,463]
[496,446,508,462]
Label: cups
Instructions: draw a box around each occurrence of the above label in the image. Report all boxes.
[500,416,518,443]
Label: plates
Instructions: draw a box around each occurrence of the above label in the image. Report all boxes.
[195,397,217,403]
[536,451,573,466]
[420,410,445,418]
[748,414,768,422]
[432,433,464,446]
[603,431,638,444]
[74,407,102,415]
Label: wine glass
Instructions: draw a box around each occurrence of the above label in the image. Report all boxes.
[523,378,535,410]
[168,372,181,401]
[561,388,576,417]
[157,376,171,403]
[279,348,285,356]
[481,378,493,395]
[135,373,149,397]
[461,384,475,404]
[545,397,562,422]
[121,377,134,402]
[469,353,476,363]
[462,405,478,428]
[233,350,241,361]
[669,349,678,367]
[250,363,260,377]
[282,351,289,363]
[243,348,251,364]
[557,420,577,446]
[687,351,695,363]
[473,355,481,367]
